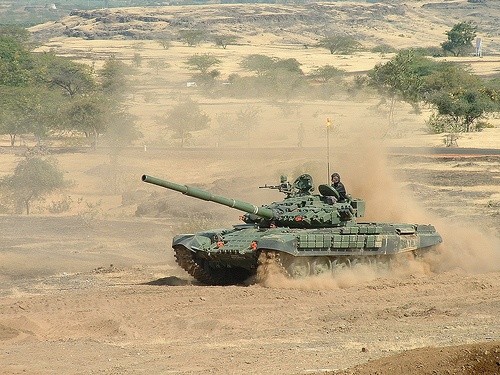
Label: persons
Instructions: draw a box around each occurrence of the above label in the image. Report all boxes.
[331,173,346,195]
[278,175,296,198]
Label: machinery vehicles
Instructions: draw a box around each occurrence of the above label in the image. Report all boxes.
[143,119,443,286]
